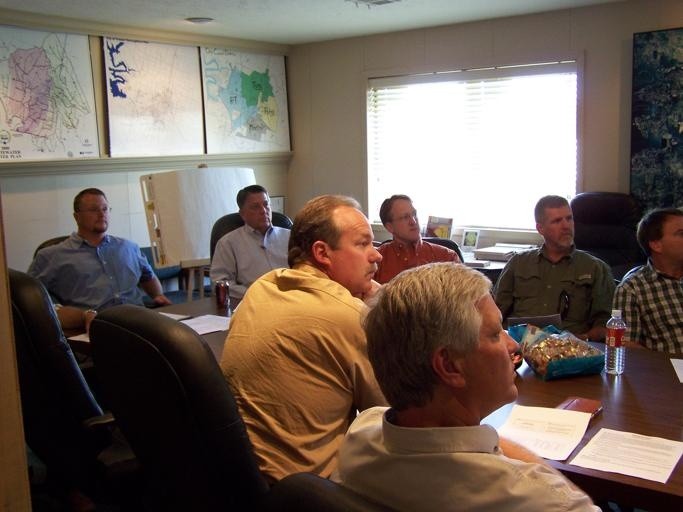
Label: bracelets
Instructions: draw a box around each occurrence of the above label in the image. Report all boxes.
[583,333,591,341]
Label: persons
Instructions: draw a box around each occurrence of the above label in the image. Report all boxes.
[329,263,604,512]
[210,185,289,299]
[372,194,458,281]
[26,188,171,330]
[219,195,390,486]
[611,209,682,354]
[493,195,616,343]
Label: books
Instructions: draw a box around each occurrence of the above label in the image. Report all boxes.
[425,216,453,241]
[462,227,479,252]
[463,260,489,266]
[473,243,537,262]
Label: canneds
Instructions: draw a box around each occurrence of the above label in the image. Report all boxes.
[215,280,231,307]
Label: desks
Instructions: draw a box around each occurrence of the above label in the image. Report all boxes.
[64,292,683,511]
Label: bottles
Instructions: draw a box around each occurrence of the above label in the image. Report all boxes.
[603,310,627,375]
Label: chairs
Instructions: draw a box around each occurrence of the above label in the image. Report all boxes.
[3,269,114,494]
[209,212,295,265]
[267,471,389,512]
[88,302,261,512]
[381,236,465,264]
[570,190,647,273]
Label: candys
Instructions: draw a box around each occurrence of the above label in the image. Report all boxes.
[521,335,601,381]
